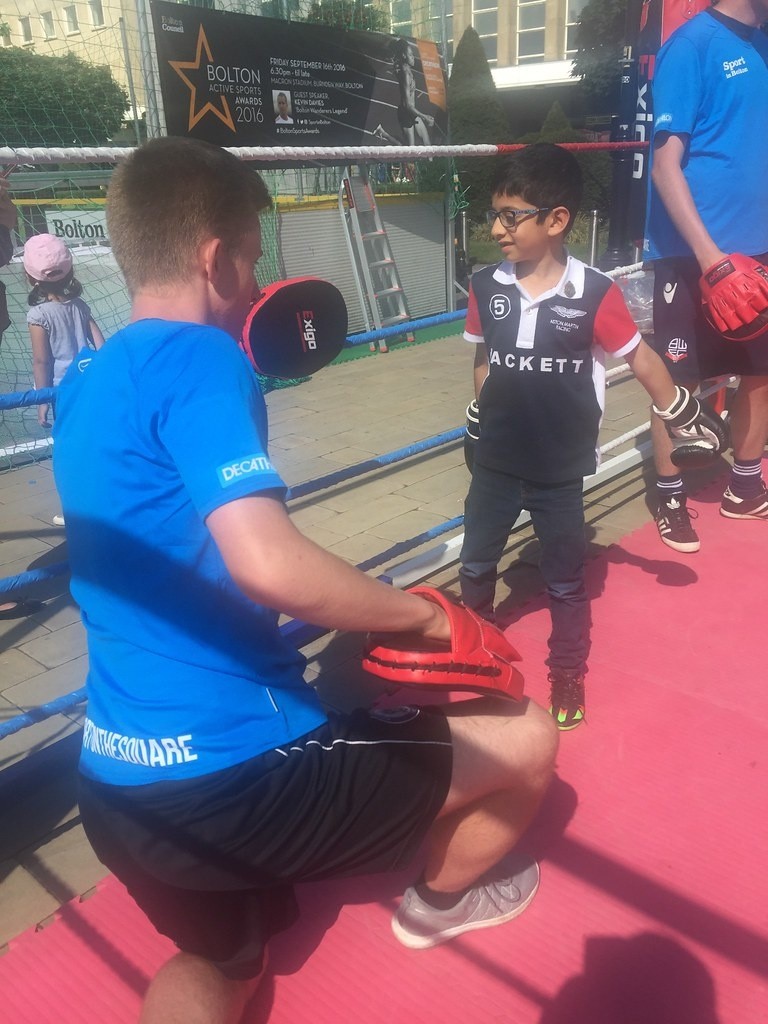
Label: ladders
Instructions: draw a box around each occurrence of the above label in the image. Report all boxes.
[336,164,416,353]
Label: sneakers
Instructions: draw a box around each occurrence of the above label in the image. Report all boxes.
[719,483,768,521]
[545,667,588,731]
[392,853,539,951]
[650,484,701,552]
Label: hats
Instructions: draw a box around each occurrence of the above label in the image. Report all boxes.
[24,233,73,284]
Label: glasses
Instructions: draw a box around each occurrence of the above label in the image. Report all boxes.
[484,209,551,230]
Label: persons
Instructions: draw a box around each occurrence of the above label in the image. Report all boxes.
[49,133,562,1023]
[456,139,733,728]
[274,92,293,124]
[0,177,18,345]
[394,38,436,162]
[21,231,107,526]
[643,1,767,554]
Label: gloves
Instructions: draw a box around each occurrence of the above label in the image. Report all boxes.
[465,397,481,474]
[652,384,730,465]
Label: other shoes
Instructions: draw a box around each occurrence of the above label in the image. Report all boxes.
[53,514,65,525]
